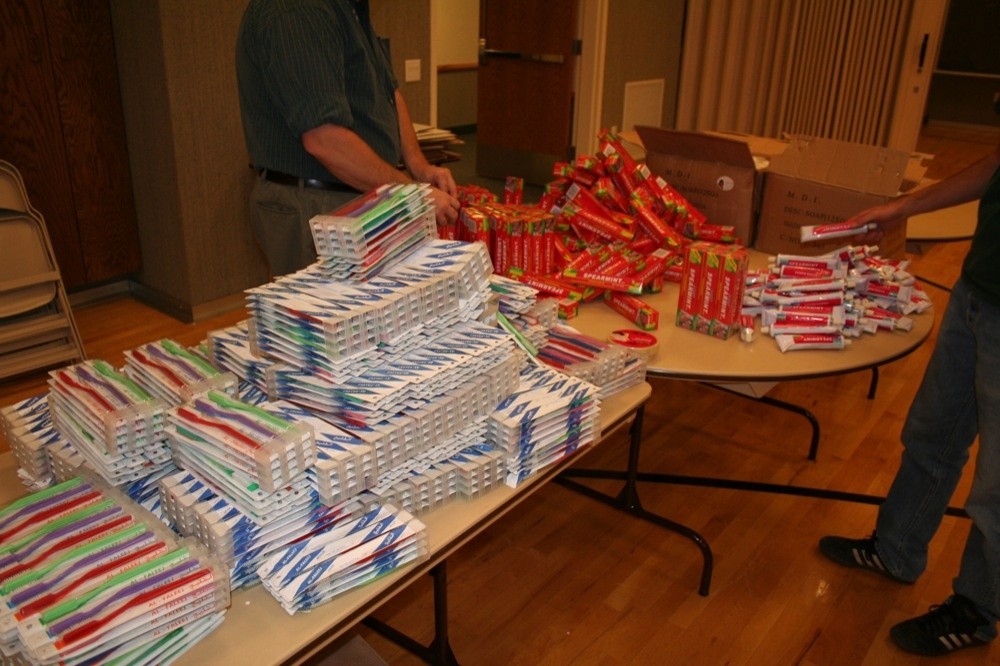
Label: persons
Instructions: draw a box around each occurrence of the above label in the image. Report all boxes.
[234,0,460,275]
[815,146,1000,656]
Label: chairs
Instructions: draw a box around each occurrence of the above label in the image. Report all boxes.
[0,156,88,384]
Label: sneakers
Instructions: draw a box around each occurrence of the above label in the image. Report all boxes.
[820,533,918,581]
[890,604,995,655]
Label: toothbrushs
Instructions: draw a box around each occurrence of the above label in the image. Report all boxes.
[0,473,233,666]
[5,180,651,614]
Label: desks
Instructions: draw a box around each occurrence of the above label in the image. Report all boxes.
[905,178,980,242]
[553,248,935,481]
[0,381,718,666]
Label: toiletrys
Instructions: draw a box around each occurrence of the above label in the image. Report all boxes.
[440,127,747,339]
[798,221,880,242]
[738,244,933,354]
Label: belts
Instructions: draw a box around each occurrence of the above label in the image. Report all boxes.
[257,165,363,194]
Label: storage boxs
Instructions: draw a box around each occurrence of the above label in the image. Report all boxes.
[756,130,934,267]
[617,123,786,248]
[437,129,749,341]
